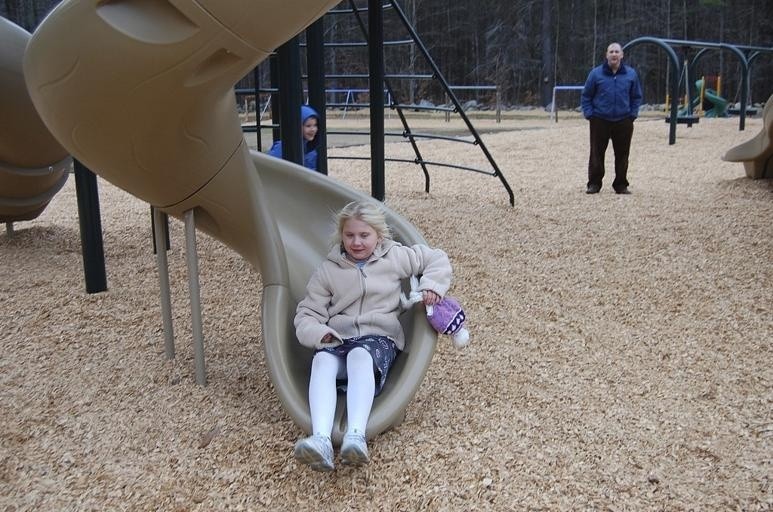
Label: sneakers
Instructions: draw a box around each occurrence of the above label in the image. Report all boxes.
[294,435,334,472]
[340,432,369,467]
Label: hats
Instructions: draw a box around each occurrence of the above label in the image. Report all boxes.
[399,275,469,347]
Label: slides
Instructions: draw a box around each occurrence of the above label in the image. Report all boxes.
[23,0,436,444]
[696,80,727,118]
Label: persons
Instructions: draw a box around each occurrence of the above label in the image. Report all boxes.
[262,104,318,171]
[581,42,643,193]
[291,198,452,475]
